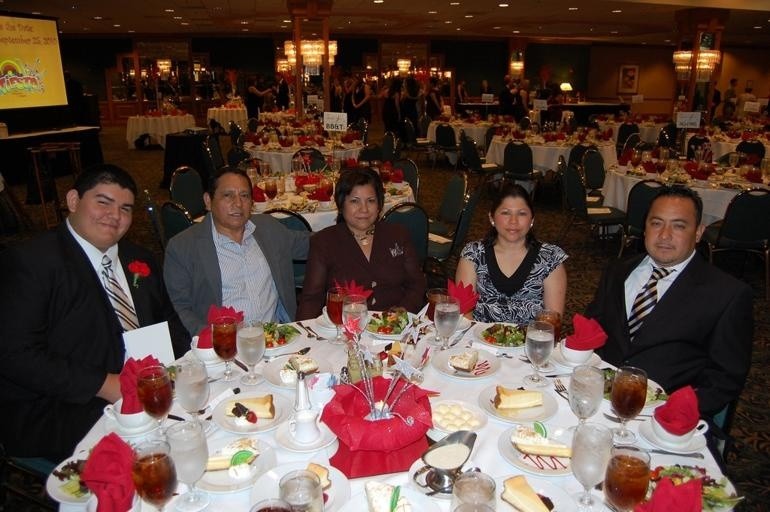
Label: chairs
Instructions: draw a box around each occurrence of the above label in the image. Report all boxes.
[147,111,496,286]
[0,441,59,511]
[702,396,741,462]
[497,110,770,280]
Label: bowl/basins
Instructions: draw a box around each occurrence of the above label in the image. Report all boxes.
[559,338,595,362]
[102,398,151,428]
[45,450,94,505]
[653,411,709,443]
[190,335,216,358]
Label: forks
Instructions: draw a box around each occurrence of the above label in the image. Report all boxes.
[298,321,316,339]
[307,326,328,343]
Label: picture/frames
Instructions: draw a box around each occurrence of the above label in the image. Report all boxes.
[616,63,640,95]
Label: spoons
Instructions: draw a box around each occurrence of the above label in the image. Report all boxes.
[426,467,481,498]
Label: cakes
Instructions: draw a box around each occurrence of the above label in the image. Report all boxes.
[450,352,479,371]
[512,425,572,458]
[289,357,318,375]
[363,481,412,512]
[206,438,257,471]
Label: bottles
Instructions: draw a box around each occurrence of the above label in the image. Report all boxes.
[449,472,497,512]
[292,371,312,411]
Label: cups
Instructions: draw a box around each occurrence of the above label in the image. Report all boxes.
[391,181,409,191]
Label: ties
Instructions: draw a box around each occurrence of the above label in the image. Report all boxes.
[629,264,676,341]
[101,255,140,333]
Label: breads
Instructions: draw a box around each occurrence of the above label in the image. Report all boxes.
[495,386,542,409]
[501,476,549,512]
[226,394,275,419]
[308,463,329,488]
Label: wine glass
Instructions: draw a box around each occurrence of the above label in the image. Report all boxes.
[423,286,461,350]
[522,321,555,387]
[236,319,267,386]
[132,440,177,512]
[213,316,238,382]
[163,420,209,512]
[444,104,591,147]
[604,444,651,512]
[628,147,750,193]
[246,162,395,212]
[247,121,349,152]
[609,367,649,444]
[608,106,762,140]
[536,308,563,347]
[324,286,369,355]
[567,422,611,512]
[171,359,209,420]
[567,365,605,433]
[139,365,173,443]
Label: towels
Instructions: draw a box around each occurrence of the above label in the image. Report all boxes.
[637,476,704,512]
[119,355,160,414]
[81,431,136,512]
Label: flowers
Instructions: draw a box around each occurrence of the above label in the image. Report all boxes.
[127,261,151,286]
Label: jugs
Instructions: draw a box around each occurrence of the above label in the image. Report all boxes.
[413,430,477,494]
[288,409,322,442]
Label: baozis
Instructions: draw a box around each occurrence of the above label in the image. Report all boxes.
[433,403,479,431]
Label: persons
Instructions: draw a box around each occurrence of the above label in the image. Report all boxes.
[482,75,560,122]
[197,73,232,100]
[164,167,298,338]
[693,78,756,131]
[247,72,289,119]
[331,72,377,127]
[455,183,570,327]
[295,166,425,322]
[127,72,191,103]
[2,163,191,468]
[382,77,444,136]
[581,184,754,468]
[455,79,467,114]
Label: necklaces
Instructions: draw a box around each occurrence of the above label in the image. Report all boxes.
[345,224,377,245]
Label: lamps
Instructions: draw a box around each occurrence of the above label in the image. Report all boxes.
[672,48,722,84]
[397,58,411,77]
[157,59,172,81]
[283,38,338,66]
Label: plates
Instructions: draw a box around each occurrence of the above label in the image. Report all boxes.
[548,350,603,369]
[255,354,322,390]
[638,422,708,454]
[605,376,668,408]
[409,457,476,503]
[648,453,738,512]
[195,437,278,492]
[365,310,418,341]
[477,384,559,423]
[210,390,291,435]
[263,321,301,348]
[272,411,338,453]
[497,425,572,479]
[429,400,489,432]
[98,409,166,439]
[472,321,526,351]
[432,348,499,381]
